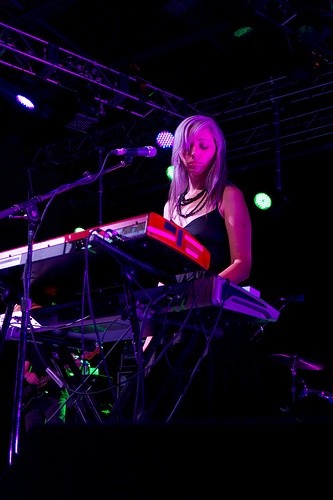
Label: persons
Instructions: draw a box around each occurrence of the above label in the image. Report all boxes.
[24,344,70,433]
[158,115,251,288]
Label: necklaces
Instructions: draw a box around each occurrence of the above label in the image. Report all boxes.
[177,186,209,219]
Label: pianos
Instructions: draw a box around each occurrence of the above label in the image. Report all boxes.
[30,277,280,349]
[0,210,213,314]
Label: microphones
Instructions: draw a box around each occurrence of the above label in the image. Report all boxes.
[111,146,157,157]
